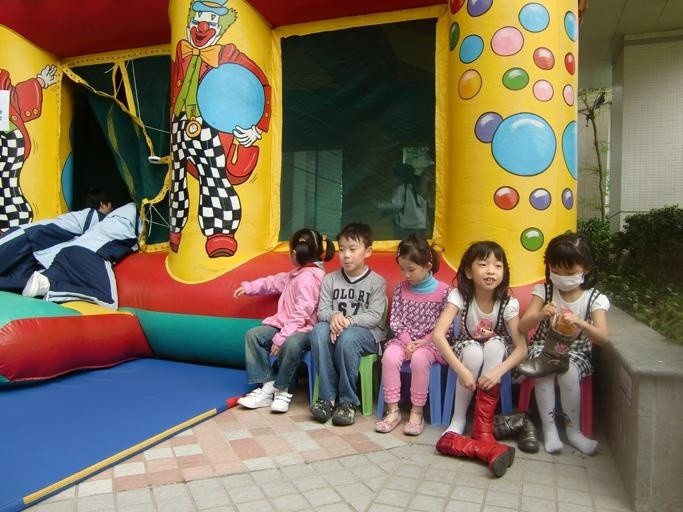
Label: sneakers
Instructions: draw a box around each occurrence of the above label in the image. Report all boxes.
[309,399,334,423]
[237,388,274,409]
[23,271,44,299]
[375,409,402,433]
[205,233,236,258]
[271,392,292,413]
[331,405,355,427]
[169,231,182,252]
[403,414,426,436]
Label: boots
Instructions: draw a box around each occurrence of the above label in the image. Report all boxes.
[439,431,516,474]
[473,383,497,445]
[516,325,569,378]
[492,412,539,452]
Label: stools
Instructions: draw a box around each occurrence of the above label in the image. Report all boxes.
[312,351,380,417]
[515,375,594,441]
[441,364,513,428]
[376,359,442,428]
[267,347,314,408]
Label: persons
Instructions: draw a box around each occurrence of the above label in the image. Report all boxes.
[0,192,119,299]
[370,163,428,239]
[430,240,529,444]
[516,232,610,455]
[20,201,143,311]
[232,229,336,413]
[373,232,462,436]
[308,221,389,426]
[414,142,436,232]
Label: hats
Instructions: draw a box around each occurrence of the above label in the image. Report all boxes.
[192,1,228,16]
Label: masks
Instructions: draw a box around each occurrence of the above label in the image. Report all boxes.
[548,272,584,292]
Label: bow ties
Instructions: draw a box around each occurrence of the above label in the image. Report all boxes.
[179,41,222,68]
